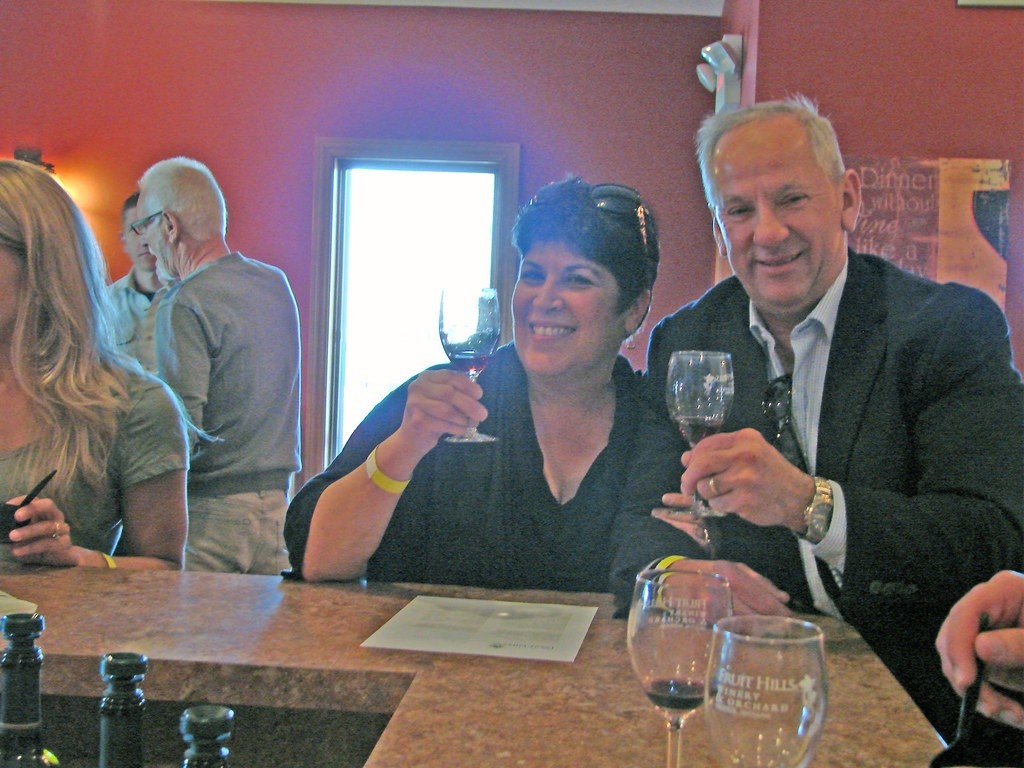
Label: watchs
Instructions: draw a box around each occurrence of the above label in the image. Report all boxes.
[794,475,835,545]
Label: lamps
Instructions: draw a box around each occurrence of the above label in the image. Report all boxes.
[695,38,740,95]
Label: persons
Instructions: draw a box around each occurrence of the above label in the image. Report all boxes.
[0,159,199,569]
[284,172,722,594]
[105,192,179,375]
[131,157,303,575]
[636,91,1024,768]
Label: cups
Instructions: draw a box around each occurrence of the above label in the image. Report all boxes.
[704,615,827,767]
[0,502,31,544]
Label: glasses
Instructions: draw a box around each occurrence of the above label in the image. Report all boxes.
[529,179,648,253]
[130,210,162,236]
[765,372,808,480]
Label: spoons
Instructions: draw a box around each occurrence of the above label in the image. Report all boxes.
[928,611,989,768]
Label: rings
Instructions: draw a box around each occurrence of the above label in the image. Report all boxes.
[51,520,60,538]
[708,476,721,498]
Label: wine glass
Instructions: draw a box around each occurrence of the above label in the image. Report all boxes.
[664,350,734,518]
[625,570,734,768]
[438,285,502,444]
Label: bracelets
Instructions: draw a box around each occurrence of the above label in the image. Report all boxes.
[99,551,117,569]
[655,555,686,572]
[367,444,410,494]
